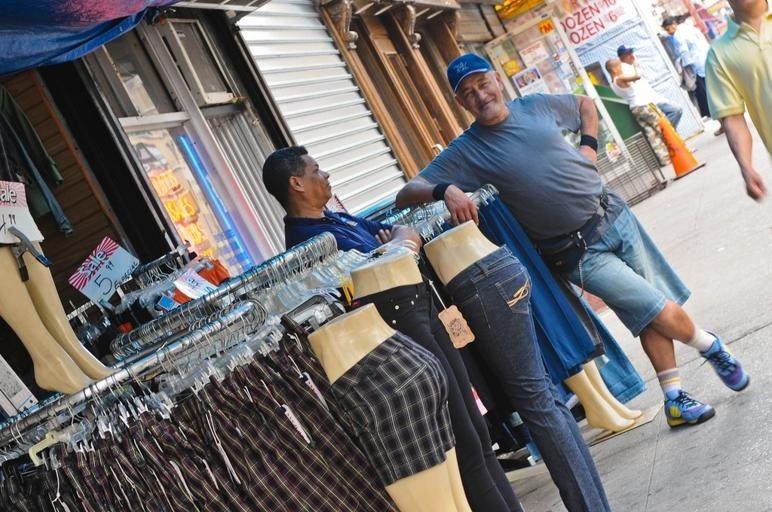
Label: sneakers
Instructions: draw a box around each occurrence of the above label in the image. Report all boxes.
[700,330,749,391]
[664,390,715,428]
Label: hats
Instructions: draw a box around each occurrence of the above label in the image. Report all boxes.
[617,44,633,56]
[447,52,493,93]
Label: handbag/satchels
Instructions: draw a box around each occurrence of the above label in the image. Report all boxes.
[534,215,601,274]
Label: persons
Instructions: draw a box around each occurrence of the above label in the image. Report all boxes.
[557,358,643,432]
[605,0,770,202]
[352,251,524,511]
[260,144,423,264]
[0,241,125,397]
[422,220,614,510]
[307,302,474,510]
[394,50,752,430]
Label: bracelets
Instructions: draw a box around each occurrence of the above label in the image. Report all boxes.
[577,136,599,151]
[432,182,452,200]
